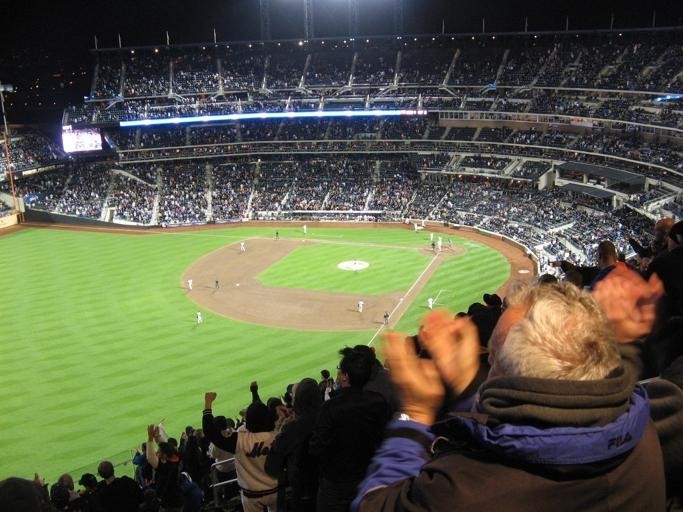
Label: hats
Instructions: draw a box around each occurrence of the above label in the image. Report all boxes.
[295,378,321,409]
